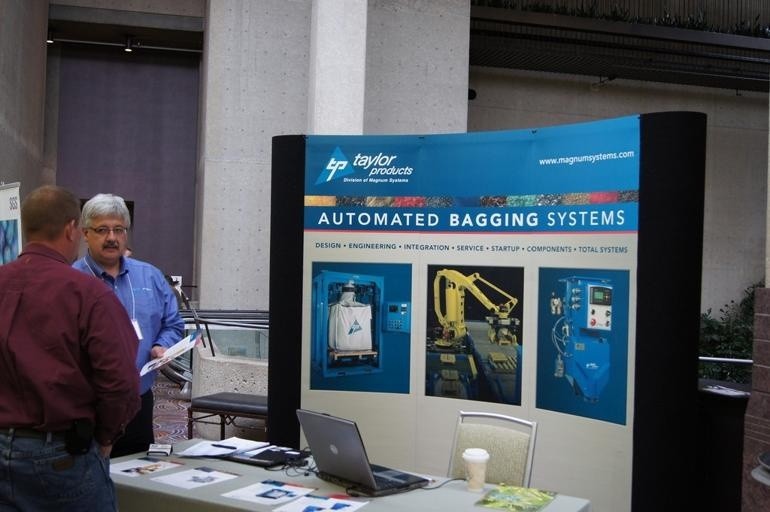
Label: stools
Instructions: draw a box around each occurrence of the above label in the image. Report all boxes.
[187,390,269,441]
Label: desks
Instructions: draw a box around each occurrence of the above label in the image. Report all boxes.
[97,437,590,512]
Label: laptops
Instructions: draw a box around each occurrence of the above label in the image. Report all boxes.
[296,408,429,498]
[213,445,311,467]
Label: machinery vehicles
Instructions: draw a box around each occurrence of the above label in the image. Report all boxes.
[430,266,520,351]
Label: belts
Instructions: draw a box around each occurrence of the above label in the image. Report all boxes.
[0,428,61,442]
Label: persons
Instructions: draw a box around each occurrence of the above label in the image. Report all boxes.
[71,194,185,458]
[164,274,183,309]
[1,183,140,511]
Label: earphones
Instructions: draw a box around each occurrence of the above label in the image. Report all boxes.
[304,471,309,476]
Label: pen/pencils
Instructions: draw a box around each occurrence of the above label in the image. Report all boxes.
[212,444,237,449]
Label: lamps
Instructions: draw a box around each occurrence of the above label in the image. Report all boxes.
[123,36,136,54]
[45,29,54,44]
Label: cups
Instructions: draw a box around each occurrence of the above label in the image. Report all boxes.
[462,446,489,492]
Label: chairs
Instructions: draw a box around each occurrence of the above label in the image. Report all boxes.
[446,411,538,489]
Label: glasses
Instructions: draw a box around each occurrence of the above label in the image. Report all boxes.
[89,226,128,234]
